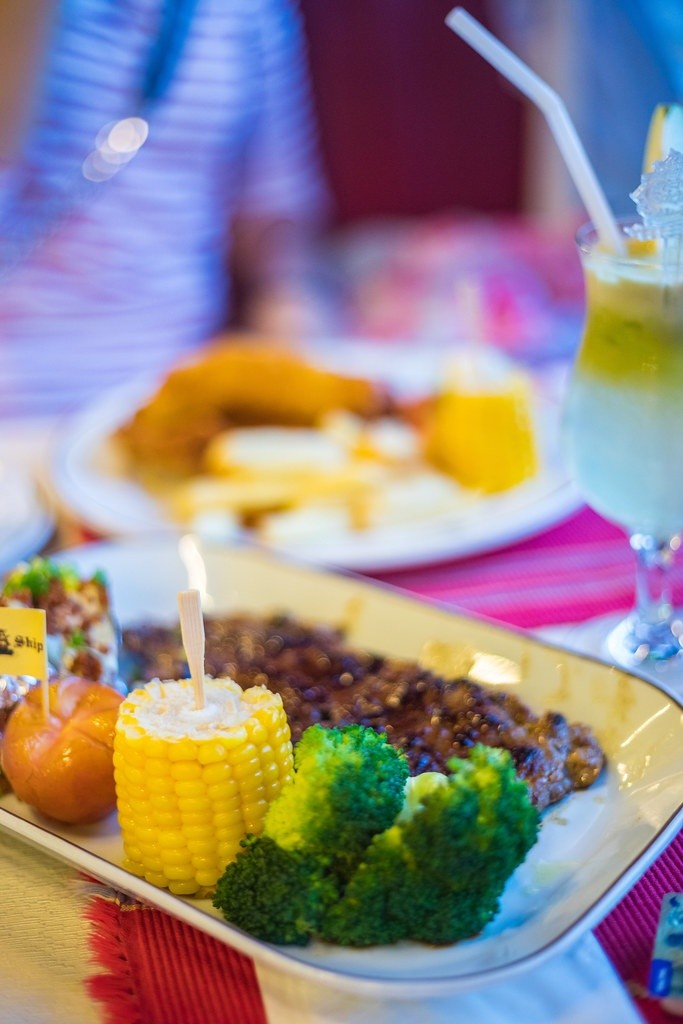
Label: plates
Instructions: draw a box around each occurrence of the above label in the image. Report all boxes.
[2,534,683,1000]
[35,326,588,578]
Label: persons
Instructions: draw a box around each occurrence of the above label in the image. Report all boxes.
[0,0,323,433]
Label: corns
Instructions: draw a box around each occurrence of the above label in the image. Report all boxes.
[112,675,296,900]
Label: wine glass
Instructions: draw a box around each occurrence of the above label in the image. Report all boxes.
[552,209,683,704]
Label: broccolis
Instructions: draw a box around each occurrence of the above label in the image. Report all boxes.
[211,723,544,950]
